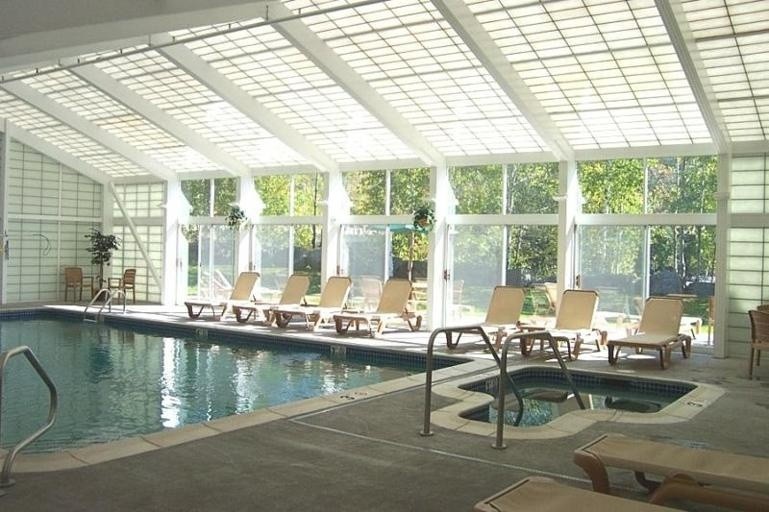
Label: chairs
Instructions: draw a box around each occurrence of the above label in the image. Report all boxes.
[627,297,702,340]
[475,477,769,512]
[518,290,608,361]
[608,295,691,367]
[65,267,93,303]
[185,272,422,339]
[709,296,715,345]
[107,269,136,304]
[446,285,531,350]
[748,305,768,380]
[574,433,769,499]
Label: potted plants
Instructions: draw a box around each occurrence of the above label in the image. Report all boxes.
[84,228,120,301]
[413,202,437,232]
